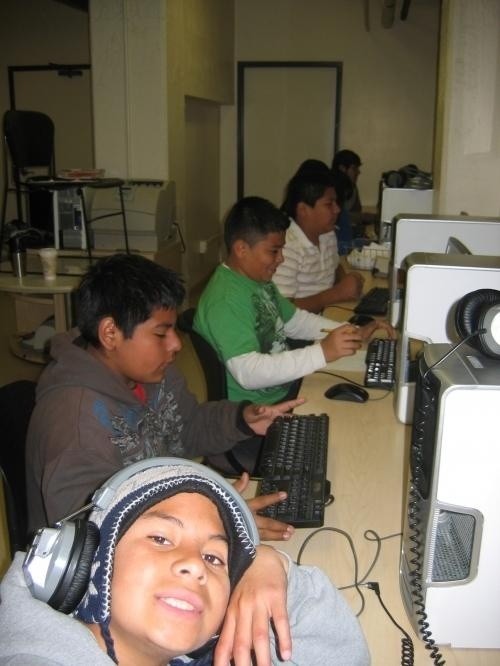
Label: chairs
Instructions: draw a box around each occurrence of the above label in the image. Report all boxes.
[0,109,129,265]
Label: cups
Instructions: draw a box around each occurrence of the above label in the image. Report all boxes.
[37,247,59,281]
[398,383,415,424]
[8,236,27,278]
[389,298,402,328]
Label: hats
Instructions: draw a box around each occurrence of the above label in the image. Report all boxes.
[65,462,260,627]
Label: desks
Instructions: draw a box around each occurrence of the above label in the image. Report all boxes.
[1,240,182,362]
[261,206,500,666]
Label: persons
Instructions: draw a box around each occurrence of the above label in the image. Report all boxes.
[275,148,365,312]
[0,457,371,665]
[24,253,306,540]
[191,196,394,468]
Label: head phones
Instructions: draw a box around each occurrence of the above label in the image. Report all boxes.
[455,286,500,359]
[24,457,261,614]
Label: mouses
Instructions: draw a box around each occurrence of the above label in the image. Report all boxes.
[323,380,370,404]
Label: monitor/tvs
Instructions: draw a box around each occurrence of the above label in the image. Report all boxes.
[443,234,474,259]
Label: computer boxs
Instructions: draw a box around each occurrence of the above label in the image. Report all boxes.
[396,342,499,649]
[390,212,500,331]
[377,185,436,243]
[395,251,500,425]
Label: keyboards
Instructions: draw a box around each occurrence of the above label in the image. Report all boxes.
[363,337,394,390]
[353,285,389,315]
[256,413,329,526]
